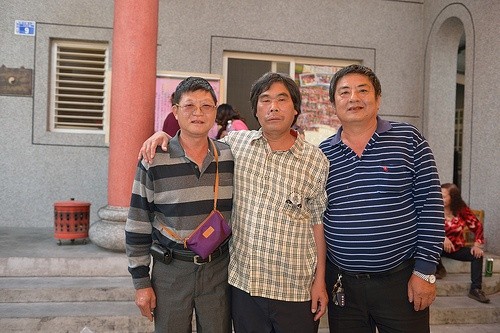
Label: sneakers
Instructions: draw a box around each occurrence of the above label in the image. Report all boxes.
[435,269,447,279]
[467,288,490,303]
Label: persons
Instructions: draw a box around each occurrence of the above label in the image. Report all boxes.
[162,92,180,138]
[137,73,330,333]
[435,183,489,302]
[214,104,248,140]
[317,65,445,333]
[125,77,235,333]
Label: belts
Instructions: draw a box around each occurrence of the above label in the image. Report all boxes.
[329,256,415,279]
[167,246,229,266]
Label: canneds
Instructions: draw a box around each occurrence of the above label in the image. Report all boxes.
[485,258,494,277]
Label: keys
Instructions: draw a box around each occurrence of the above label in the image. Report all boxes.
[332,275,345,308]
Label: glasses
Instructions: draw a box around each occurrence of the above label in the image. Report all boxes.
[175,103,216,114]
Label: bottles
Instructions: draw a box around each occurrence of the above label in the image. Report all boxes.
[227,120,234,134]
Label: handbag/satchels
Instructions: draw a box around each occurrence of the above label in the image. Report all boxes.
[184,210,232,260]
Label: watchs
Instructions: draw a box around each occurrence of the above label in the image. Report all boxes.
[413,270,437,285]
